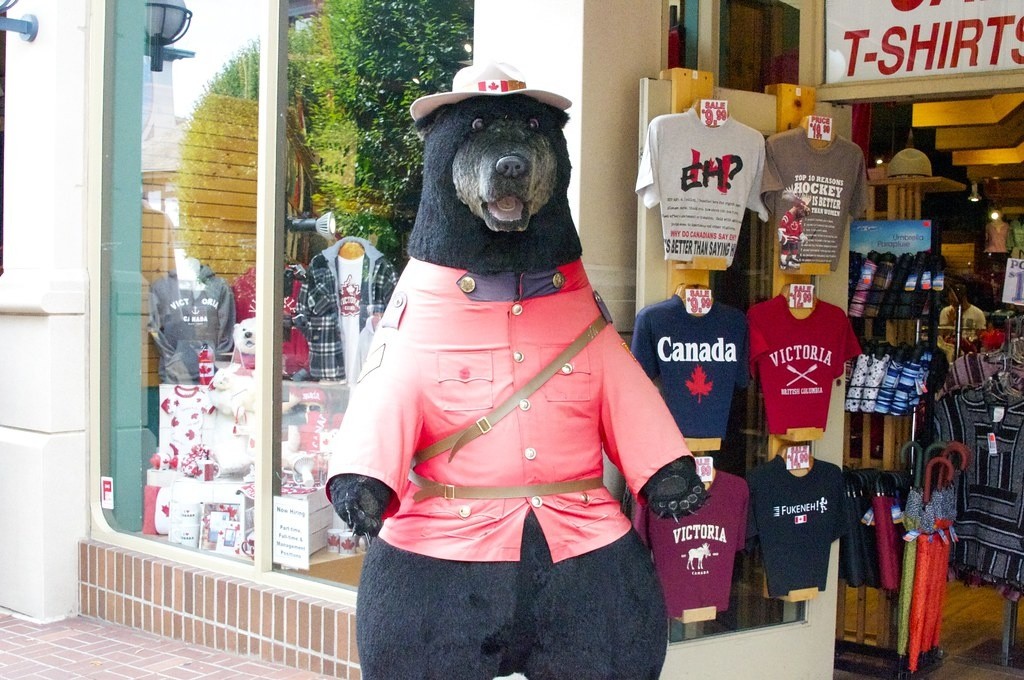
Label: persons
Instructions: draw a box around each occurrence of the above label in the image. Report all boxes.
[289,234,399,384]
[938,282,988,358]
[146,255,237,386]
[230,263,312,382]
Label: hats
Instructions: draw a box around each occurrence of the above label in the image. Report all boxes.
[409,61,573,141]
[288,386,324,404]
[887,147,933,179]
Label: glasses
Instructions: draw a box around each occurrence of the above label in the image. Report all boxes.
[293,404,320,414]
